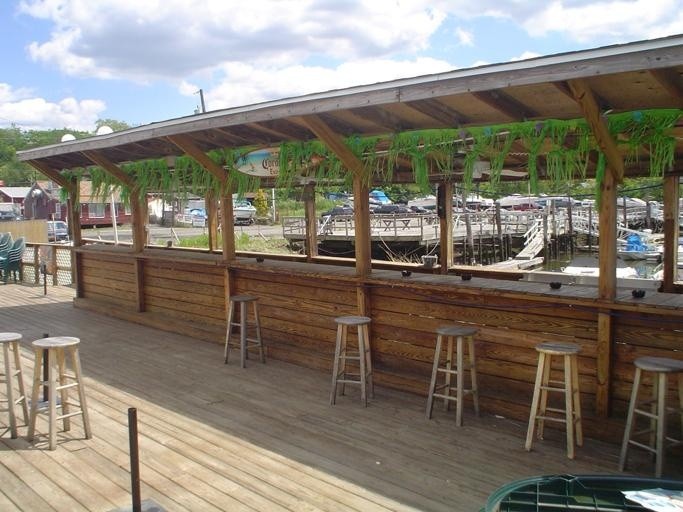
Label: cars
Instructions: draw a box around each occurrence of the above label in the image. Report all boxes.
[46,221,68,241]
[0,212,29,221]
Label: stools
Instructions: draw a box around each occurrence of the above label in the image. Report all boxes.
[26,335,94,451]
[223,294,266,369]
[617,355,683,479]
[524,341,584,460]
[330,315,375,409]
[425,325,482,428]
[0,332,30,441]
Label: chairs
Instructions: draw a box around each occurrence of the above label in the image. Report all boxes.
[0,231,27,286]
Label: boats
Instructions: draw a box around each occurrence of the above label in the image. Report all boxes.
[187,208,207,220]
[232,199,256,226]
[617,237,683,281]
[321,191,683,236]
